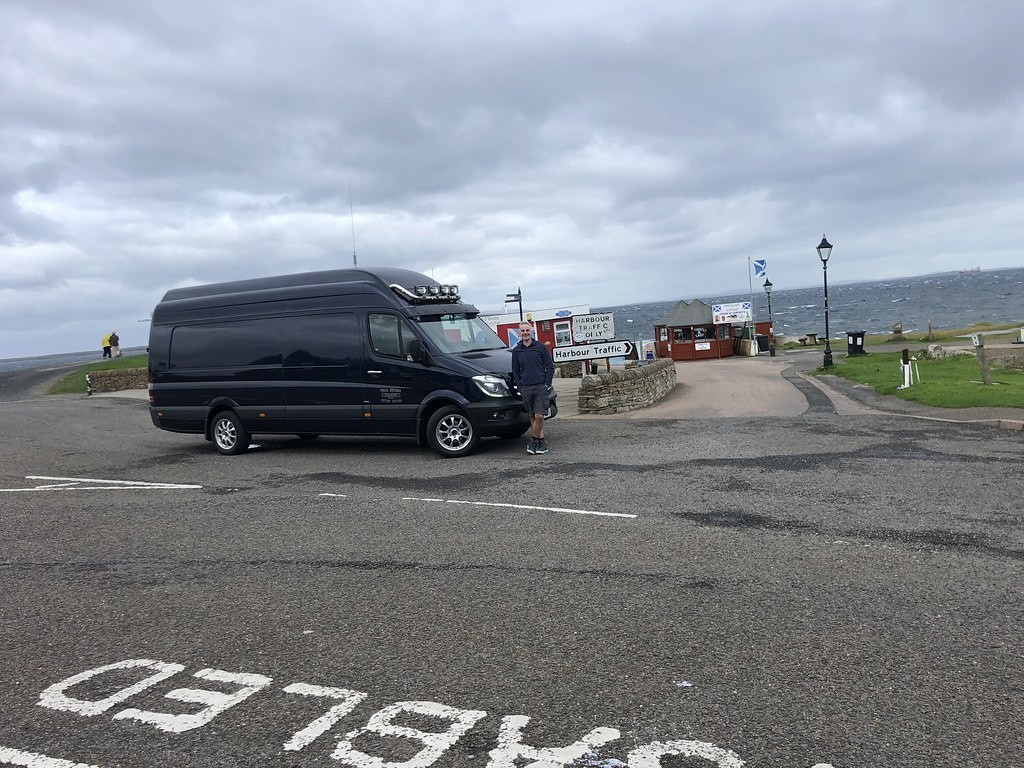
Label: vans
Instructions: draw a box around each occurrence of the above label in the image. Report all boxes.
[146,266,561,456]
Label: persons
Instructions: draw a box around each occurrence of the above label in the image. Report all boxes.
[110,331,121,358]
[512,320,556,454]
[101,334,113,359]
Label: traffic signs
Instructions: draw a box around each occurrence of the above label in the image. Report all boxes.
[552,339,634,364]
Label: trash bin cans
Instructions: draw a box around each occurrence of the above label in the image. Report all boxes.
[846,330,867,355]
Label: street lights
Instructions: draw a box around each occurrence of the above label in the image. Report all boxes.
[815,233,835,368]
[762,278,776,356]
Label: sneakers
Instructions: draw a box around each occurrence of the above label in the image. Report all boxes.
[527,439,539,454]
[535,441,548,453]
